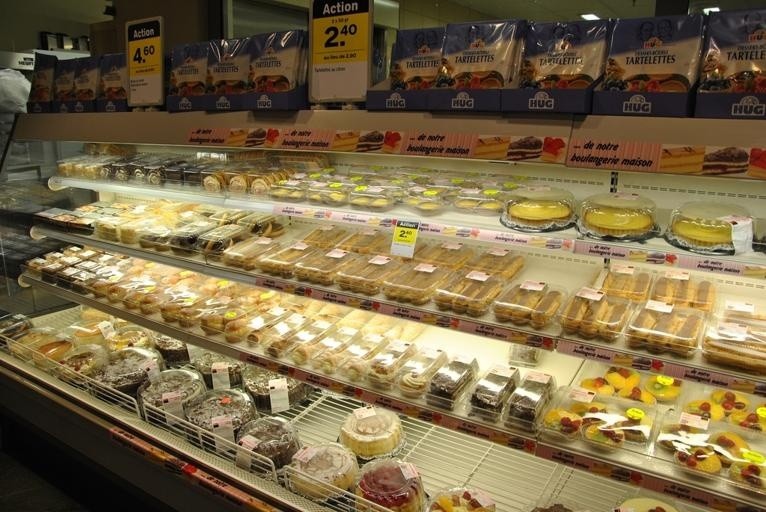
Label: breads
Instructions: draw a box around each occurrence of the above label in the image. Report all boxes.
[602,300,629,339]
[529,289,560,327]
[654,271,674,304]
[579,297,607,340]
[646,308,683,352]
[496,283,523,320]
[628,271,652,301]
[221,224,389,296]
[514,284,543,324]
[561,293,591,334]
[591,267,612,293]
[609,272,632,297]
[497,254,523,281]
[632,303,657,340]
[390,238,496,317]
[675,273,694,306]
[671,313,701,353]
[696,279,715,309]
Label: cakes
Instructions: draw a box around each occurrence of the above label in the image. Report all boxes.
[645,373,684,402]
[338,405,405,464]
[502,371,553,431]
[618,386,658,406]
[577,193,662,240]
[471,363,521,422]
[581,418,622,450]
[151,331,196,367]
[500,186,578,232]
[725,460,766,496]
[97,257,179,324]
[381,130,400,152]
[472,136,510,160]
[183,386,258,453]
[33,340,72,371]
[0,313,28,347]
[659,145,705,173]
[709,431,751,465]
[701,146,750,174]
[277,164,451,212]
[570,400,605,420]
[60,155,294,199]
[541,136,566,162]
[241,285,321,363]
[288,440,354,506]
[543,406,581,440]
[13,329,63,359]
[702,300,765,371]
[314,303,412,385]
[606,365,640,389]
[88,344,163,404]
[193,347,245,391]
[105,327,154,351]
[726,411,766,437]
[265,127,281,148]
[58,346,105,382]
[332,132,356,151]
[686,399,722,424]
[428,486,496,511]
[225,131,246,147]
[507,342,539,368]
[581,378,613,396]
[240,362,311,411]
[660,419,709,450]
[244,128,265,147]
[357,131,382,151]
[72,320,112,345]
[425,358,479,408]
[616,412,654,446]
[173,275,246,341]
[396,346,447,399]
[353,455,428,511]
[234,416,299,473]
[711,387,749,415]
[136,367,209,424]
[25,243,120,292]
[80,196,281,263]
[665,200,752,254]
[506,135,543,159]
[673,445,721,480]
[453,176,524,212]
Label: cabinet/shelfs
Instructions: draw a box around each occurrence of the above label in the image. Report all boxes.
[0,138,765,512]
